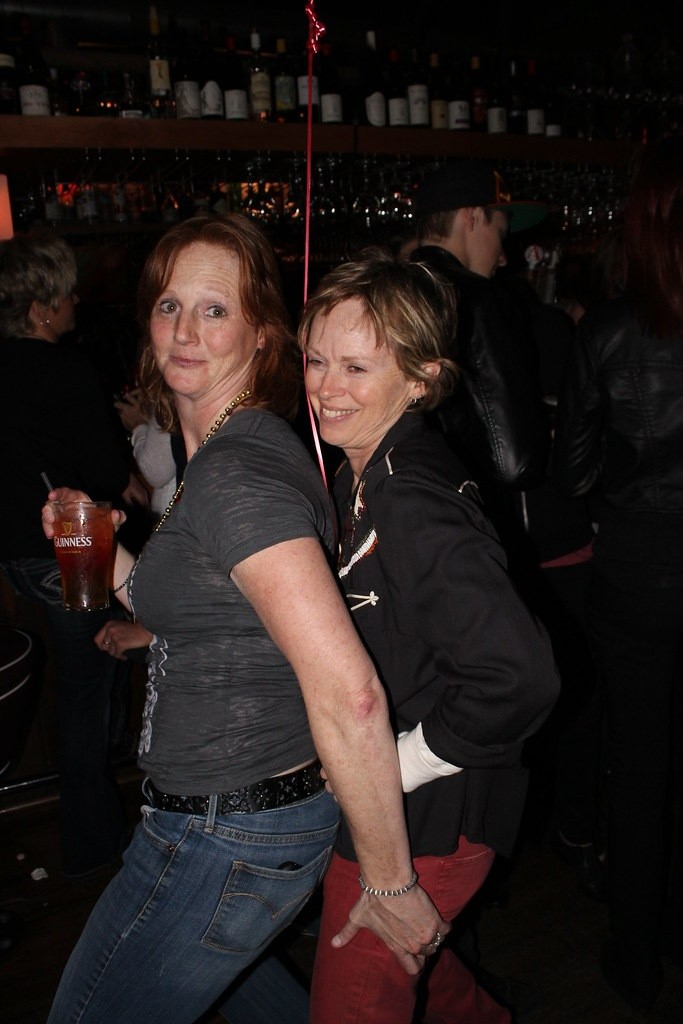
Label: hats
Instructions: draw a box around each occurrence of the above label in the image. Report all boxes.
[414,155,551,234]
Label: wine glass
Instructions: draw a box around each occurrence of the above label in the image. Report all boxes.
[0,145,628,243]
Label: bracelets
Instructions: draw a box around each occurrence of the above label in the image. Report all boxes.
[358,872,418,896]
[113,559,138,593]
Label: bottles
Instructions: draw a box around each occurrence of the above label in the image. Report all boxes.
[0,7,683,136]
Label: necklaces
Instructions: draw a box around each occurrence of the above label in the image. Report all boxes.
[155,388,252,531]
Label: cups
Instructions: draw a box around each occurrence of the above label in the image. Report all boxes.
[52,500,114,611]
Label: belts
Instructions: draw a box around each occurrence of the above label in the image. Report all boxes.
[147,757,327,816]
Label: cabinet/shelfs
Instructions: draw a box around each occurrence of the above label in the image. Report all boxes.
[0,0,683,284]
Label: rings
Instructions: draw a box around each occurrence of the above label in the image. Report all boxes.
[430,933,441,948]
[103,640,111,644]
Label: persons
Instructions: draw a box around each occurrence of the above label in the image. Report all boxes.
[0,237,296,869]
[46,213,452,1024]
[393,163,682,1008]
[297,256,560,1024]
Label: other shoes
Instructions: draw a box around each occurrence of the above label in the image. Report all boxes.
[102,731,140,763]
[62,828,135,877]
[599,960,664,1020]
